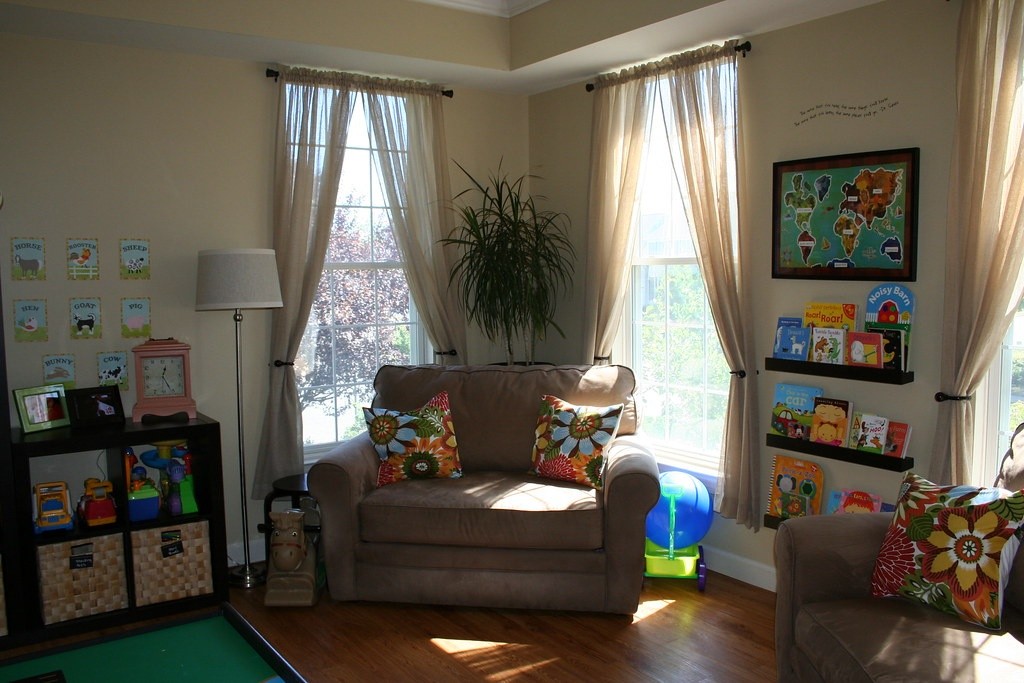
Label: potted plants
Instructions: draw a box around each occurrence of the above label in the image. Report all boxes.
[428,156,581,366]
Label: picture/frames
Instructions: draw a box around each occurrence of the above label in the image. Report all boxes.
[65,383,126,427]
[771,146,921,281]
[12,383,71,434]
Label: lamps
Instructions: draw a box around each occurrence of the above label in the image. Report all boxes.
[194,248,284,589]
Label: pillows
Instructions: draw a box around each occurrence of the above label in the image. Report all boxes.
[869,471,1024,631]
[526,395,625,492]
[363,391,462,487]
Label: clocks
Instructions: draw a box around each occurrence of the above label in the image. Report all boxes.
[131,337,196,422]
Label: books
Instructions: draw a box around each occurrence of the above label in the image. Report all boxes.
[772,301,911,372]
[766,384,913,518]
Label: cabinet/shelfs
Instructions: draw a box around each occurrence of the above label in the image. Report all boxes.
[0,410,228,637]
[764,357,914,528]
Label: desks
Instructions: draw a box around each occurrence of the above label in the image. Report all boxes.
[0,602,310,682]
[260,475,326,591]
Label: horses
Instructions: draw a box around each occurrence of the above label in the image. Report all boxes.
[267,508,316,606]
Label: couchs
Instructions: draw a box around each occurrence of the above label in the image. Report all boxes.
[307,364,661,616]
[772,420,1024,683]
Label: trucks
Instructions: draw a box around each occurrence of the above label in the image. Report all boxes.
[32,481,75,534]
